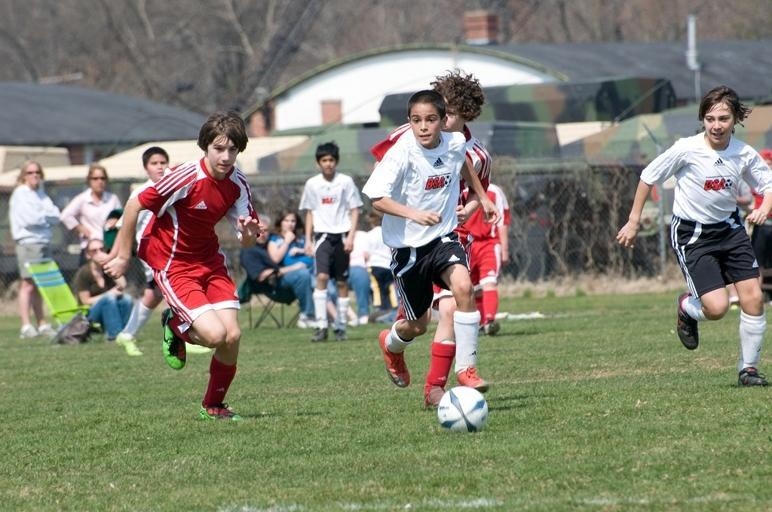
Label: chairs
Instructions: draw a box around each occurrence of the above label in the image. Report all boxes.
[236,243,307,330]
[20,255,95,349]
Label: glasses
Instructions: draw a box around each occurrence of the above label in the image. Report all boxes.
[26,171,42,174]
[91,176,108,181]
[90,247,105,254]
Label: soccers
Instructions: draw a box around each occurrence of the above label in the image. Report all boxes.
[438,387,487,432]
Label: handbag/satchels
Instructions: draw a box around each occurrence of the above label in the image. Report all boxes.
[51,310,93,346]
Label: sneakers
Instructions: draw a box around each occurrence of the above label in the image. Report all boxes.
[455,366,491,395]
[738,365,769,388]
[200,400,245,423]
[424,383,450,410]
[334,328,349,342]
[483,318,501,336]
[377,329,412,388]
[311,327,329,343]
[159,307,187,371]
[676,291,701,351]
[19,322,39,339]
[37,323,59,336]
[115,332,144,357]
